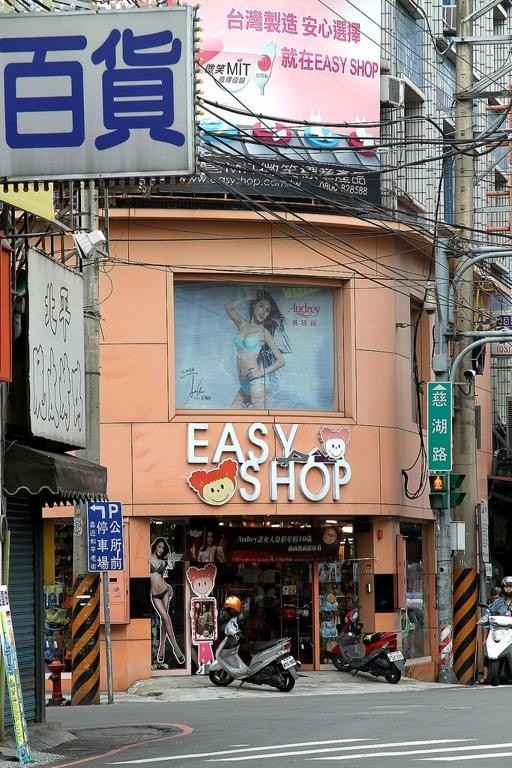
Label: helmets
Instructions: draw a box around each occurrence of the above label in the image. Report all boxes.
[224,595,241,613]
[499,576,512,598]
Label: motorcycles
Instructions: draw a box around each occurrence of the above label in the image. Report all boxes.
[208,615,300,692]
[330,605,406,685]
[476,600,512,685]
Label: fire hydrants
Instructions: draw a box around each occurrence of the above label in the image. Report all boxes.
[49,658,66,703]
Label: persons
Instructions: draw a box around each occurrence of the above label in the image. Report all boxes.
[482,587,501,649]
[198,604,211,636]
[224,290,286,411]
[198,530,227,563]
[146,537,185,665]
[479,575,511,682]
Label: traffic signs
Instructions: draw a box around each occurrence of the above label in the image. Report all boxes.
[426,382,454,471]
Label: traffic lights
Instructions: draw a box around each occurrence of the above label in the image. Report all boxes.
[429,474,447,509]
[450,473,467,507]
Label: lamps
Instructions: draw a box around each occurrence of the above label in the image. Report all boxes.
[71,227,108,263]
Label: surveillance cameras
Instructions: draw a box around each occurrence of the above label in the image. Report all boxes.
[424,294,437,315]
[463,370,477,384]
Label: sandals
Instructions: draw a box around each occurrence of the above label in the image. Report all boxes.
[158,651,163,662]
[475,678,490,685]
[175,651,184,663]
